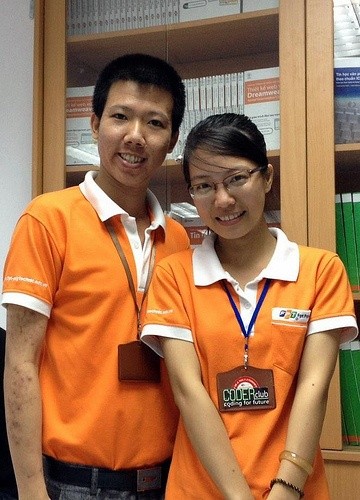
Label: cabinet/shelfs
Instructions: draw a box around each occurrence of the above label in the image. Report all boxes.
[32,0,360,500]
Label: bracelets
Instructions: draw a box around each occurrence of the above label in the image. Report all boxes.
[278,450,314,476]
[262,479,305,497]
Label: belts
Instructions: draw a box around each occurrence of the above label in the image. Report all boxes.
[44,456,171,494]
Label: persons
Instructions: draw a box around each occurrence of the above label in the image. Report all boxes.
[0,53,194,500]
[138,112,358,500]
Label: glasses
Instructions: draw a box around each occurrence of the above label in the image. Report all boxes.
[187,166,264,199]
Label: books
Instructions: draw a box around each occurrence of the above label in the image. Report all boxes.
[335,192,360,294]
[336,341,360,448]
[65,0,280,38]
[170,200,280,253]
[333,0,360,147]
[65,64,282,162]
